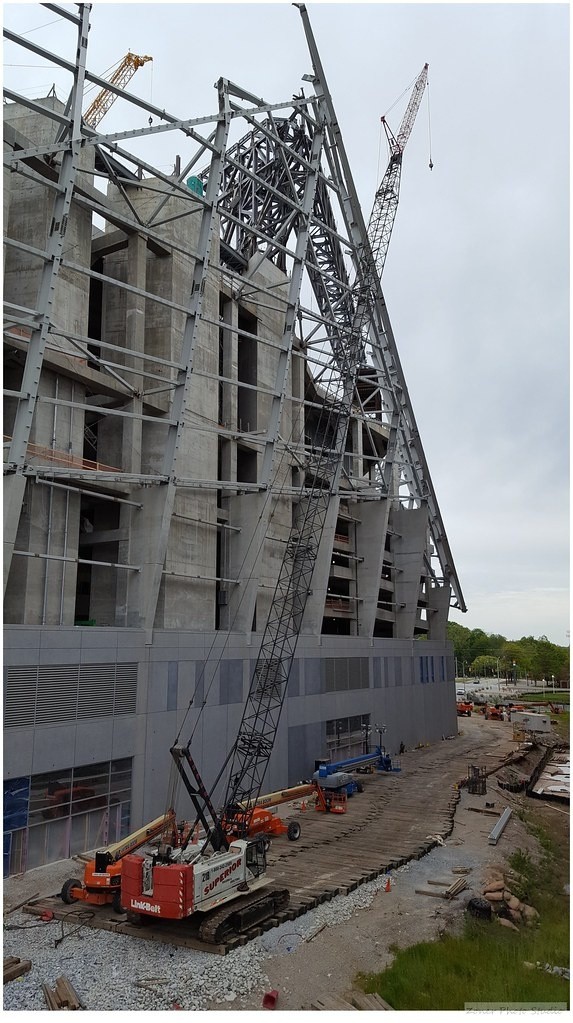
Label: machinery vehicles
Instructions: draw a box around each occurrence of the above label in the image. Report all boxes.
[214,783,313,854]
[456,679,564,725]
[61,808,183,914]
[120,60,437,952]
[312,748,389,798]
[41,778,108,820]
[308,778,348,814]
